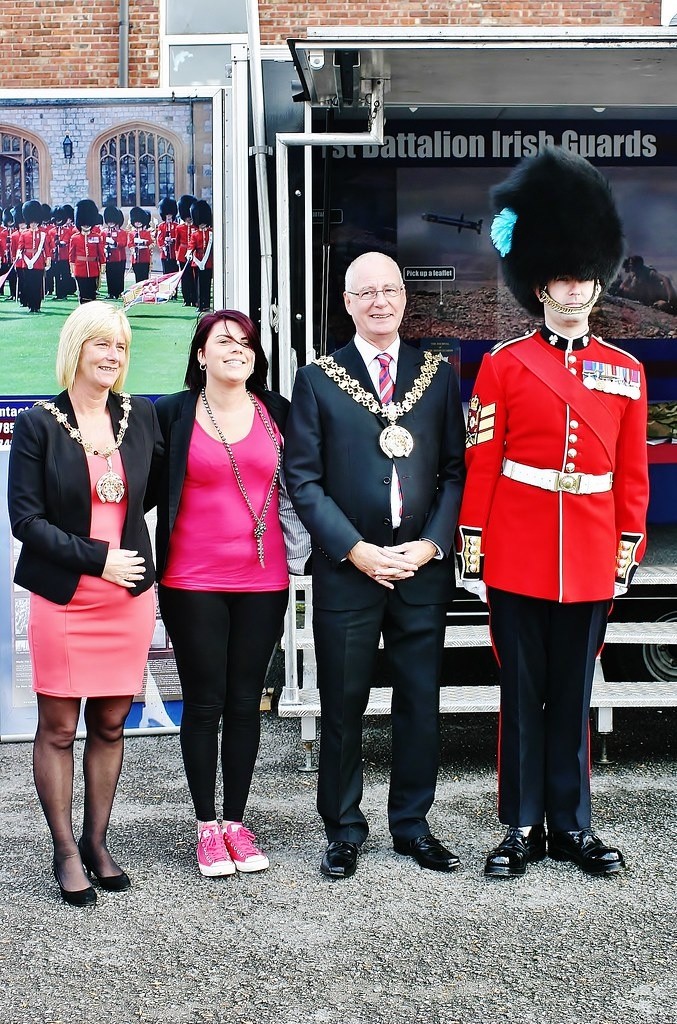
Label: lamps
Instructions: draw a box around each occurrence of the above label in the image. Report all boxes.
[63,133,74,160]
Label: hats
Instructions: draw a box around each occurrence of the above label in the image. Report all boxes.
[0,195,214,233]
[489,144,627,309]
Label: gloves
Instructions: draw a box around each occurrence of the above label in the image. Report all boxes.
[106,236,115,245]
[187,254,193,261]
[613,583,628,600]
[463,578,487,603]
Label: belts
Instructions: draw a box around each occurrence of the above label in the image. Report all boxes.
[136,246,148,249]
[105,245,117,248]
[503,457,613,494]
[60,240,66,244]
[23,248,38,253]
[77,255,99,262]
[179,243,187,247]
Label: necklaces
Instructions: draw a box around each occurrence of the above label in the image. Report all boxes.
[202,387,281,568]
[33,391,132,503]
[311,350,441,458]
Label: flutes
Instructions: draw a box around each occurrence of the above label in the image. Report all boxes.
[103,225,115,263]
[134,225,141,264]
[5,223,12,267]
[165,219,172,261]
[55,225,61,265]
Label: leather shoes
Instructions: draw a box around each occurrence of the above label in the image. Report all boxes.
[393,831,459,870]
[321,839,358,878]
[484,823,547,877]
[547,824,626,874]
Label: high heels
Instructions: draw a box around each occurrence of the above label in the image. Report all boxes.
[77,835,129,892]
[54,858,97,906]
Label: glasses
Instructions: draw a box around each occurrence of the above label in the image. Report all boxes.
[347,282,407,301]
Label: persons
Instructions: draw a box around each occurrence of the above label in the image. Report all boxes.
[8,300,165,907]
[456,273,650,878]
[0,196,214,316]
[284,253,467,876]
[145,309,313,877]
[620,256,677,309]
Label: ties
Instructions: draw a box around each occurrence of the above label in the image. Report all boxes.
[375,352,404,520]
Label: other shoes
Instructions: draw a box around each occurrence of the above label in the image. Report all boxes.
[0,287,211,313]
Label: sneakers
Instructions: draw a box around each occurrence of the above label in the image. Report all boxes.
[222,822,269,873]
[197,824,235,877]
